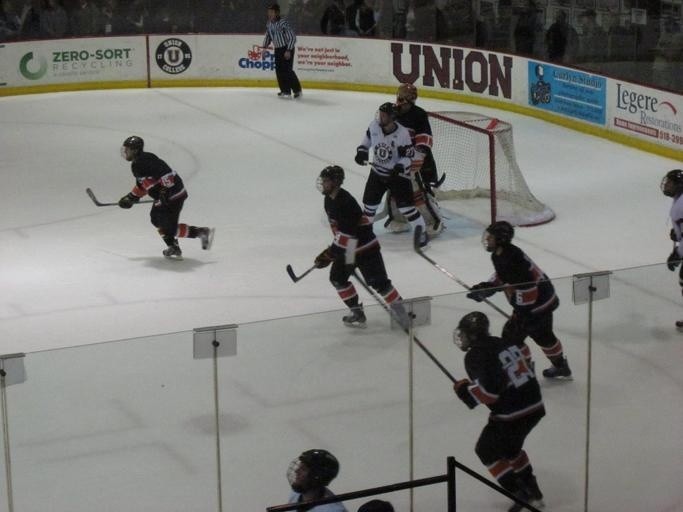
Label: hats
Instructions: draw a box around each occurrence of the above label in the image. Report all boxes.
[265,4,279,11]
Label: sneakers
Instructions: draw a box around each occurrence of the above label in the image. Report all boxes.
[507,482,543,512]
[419,231,429,246]
[163,247,181,255]
[197,227,208,249]
[278,89,301,98]
[342,311,366,323]
[543,360,571,377]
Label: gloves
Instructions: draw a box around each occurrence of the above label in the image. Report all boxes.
[155,188,168,206]
[355,145,368,165]
[509,311,528,332]
[467,282,495,301]
[453,379,477,409]
[391,164,404,177]
[315,249,334,269]
[668,252,680,270]
[119,194,139,208]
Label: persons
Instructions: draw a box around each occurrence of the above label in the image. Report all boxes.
[282,448,347,512]
[0,0,256,40]
[451,310,547,511]
[257,3,301,99]
[290,0,607,71]
[660,169,682,332]
[115,135,211,260]
[313,166,405,323]
[384,81,438,231]
[354,102,427,249]
[465,221,573,379]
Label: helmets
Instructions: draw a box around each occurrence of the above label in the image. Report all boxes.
[121,136,144,160]
[397,84,416,105]
[453,311,488,351]
[317,166,344,194]
[661,170,683,196]
[288,449,338,494]
[482,221,514,251]
[377,102,398,126]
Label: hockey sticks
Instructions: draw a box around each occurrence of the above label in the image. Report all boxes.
[86,188,158,207]
[374,188,390,221]
[287,262,321,283]
[363,159,446,188]
[413,224,512,320]
[328,247,458,384]
